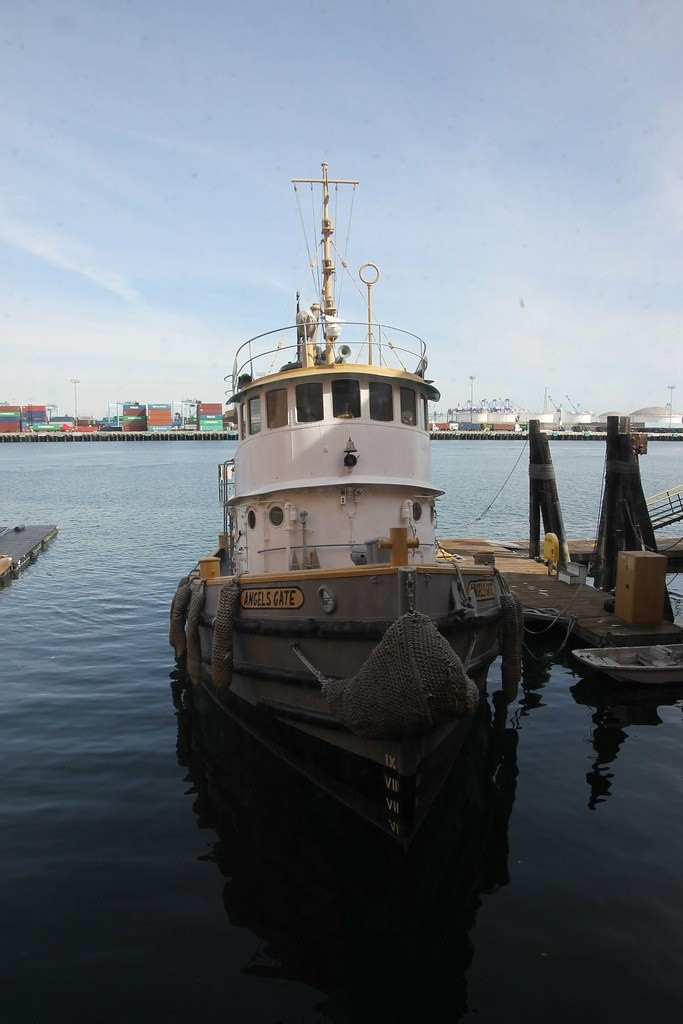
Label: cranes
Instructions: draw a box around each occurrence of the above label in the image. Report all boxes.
[457,394,587,415]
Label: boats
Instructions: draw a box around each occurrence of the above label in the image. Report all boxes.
[166,164,530,848]
[570,643,683,687]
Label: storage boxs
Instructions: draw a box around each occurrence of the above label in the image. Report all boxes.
[429,422,528,432]
[0,403,234,433]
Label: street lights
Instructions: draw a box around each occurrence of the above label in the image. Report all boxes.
[468,376,477,424]
[667,385,677,428]
[70,379,81,417]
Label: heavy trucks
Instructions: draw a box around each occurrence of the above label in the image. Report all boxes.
[33,424,72,432]
[429,423,450,431]
